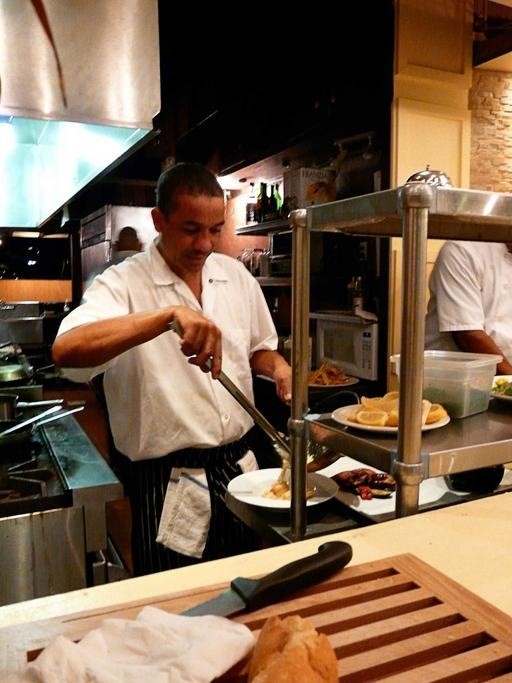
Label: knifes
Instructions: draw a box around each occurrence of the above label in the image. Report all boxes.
[173,539,353,620]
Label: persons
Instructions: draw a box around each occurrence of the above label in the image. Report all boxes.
[425,235,512,377]
[81,223,148,499]
[50,162,297,577]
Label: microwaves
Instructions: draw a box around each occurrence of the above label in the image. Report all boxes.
[316,320,379,383]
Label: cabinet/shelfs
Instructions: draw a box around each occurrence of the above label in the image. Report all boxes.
[233,216,292,288]
[286,178,512,536]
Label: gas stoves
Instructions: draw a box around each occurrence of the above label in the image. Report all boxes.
[1,344,124,561]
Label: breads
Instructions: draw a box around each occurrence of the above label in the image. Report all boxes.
[247,615,338,683]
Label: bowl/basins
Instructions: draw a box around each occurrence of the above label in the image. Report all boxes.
[44,311,54,315]
[445,466,506,494]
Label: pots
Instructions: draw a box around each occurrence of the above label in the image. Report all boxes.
[0,338,85,445]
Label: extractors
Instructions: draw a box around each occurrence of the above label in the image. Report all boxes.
[0,0,161,233]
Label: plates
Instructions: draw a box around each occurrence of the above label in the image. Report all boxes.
[331,405,452,437]
[490,374,512,399]
[226,466,338,513]
[310,375,361,391]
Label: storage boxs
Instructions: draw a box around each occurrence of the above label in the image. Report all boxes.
[282,167,337,216]
[389,347,505,420]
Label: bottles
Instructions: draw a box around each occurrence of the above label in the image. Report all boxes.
[347,277,364,312]
[239,176,292,281]
[278,334,292,363]
[63,298,70,311]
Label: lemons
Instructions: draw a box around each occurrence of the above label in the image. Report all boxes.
[348,390,443,426]
